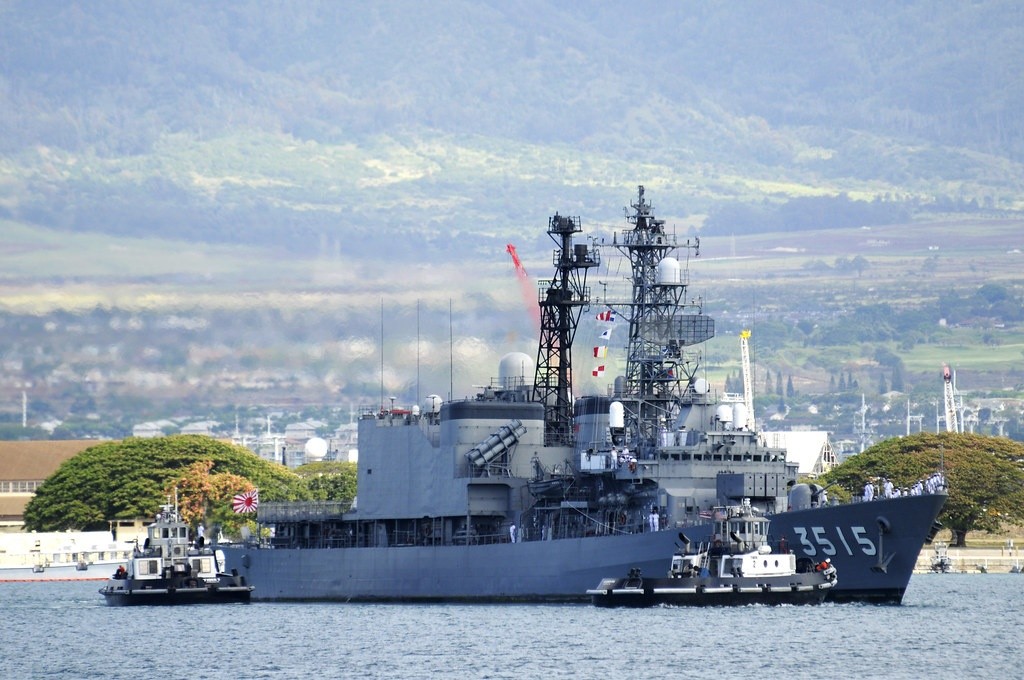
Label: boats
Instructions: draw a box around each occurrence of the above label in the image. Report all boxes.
[582,493,841,609]
[202,184,952,607]
[95,485,256,606]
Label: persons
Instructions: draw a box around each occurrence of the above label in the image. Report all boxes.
[648,510,660,532]
[509,522,516,543]
[197,522,205,537]
[863,472,944,502]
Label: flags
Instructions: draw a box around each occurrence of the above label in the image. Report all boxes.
[596,310,617,322]
[232,488,258,513]
[592,365,605,377]
[593,346,608,358]
[598,328,612,340]
